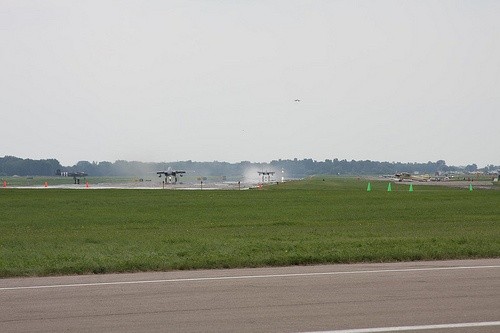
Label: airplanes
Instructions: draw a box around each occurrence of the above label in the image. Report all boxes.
[157,166,186,183]
[257,170,275,182]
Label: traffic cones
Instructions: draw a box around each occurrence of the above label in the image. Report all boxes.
[469,183,473,191]
[408,183,413,192]
[387,182,391,192]
[367,182,371,191]
[3,180,7,187]
[44,181,48,187]
[85,181,89,186]
